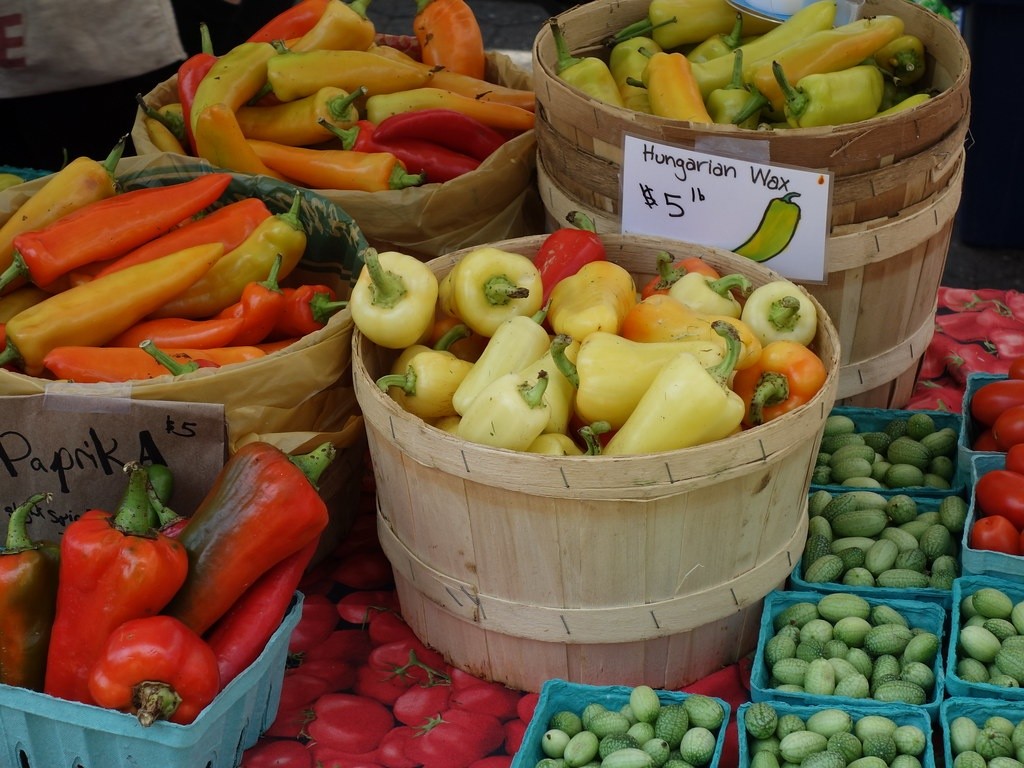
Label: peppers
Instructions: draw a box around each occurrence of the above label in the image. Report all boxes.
[0,0,950,725]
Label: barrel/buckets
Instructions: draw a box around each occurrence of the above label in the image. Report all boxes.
[348,229,840,694]
[129,32,536,262]
[0,151,369,573]
[533,0,972,410]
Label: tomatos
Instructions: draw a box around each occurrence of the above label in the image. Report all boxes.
[970,356,1024,556]
[231,288,1023,766]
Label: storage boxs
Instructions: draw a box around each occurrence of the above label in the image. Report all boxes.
[733,700,936,768]
[1,589,308,768]
[956,451,1024,585]
[956,369,1022,498]
[938,694,1022,768]
[808,403,963,500]
[942,570,1023,700]
[504,676,732,768]
[787,483,967,613]
[749,589,948,714]
